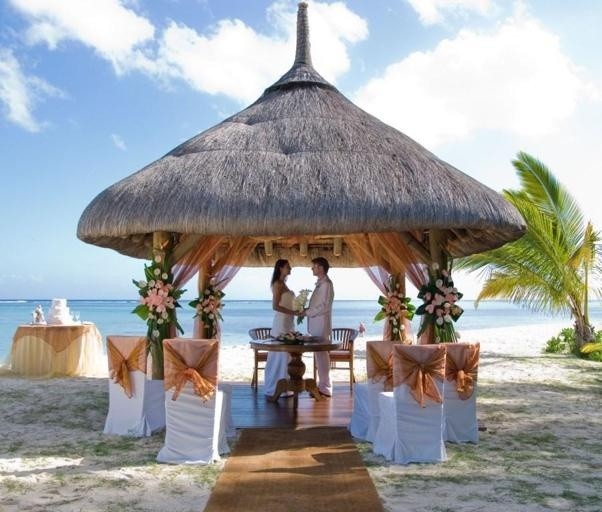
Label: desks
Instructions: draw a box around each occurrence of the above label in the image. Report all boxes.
[246,334,344,427]
[12,322,99,380]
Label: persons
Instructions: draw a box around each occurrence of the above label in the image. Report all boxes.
[263,258,300,397]
[296,257,334,397]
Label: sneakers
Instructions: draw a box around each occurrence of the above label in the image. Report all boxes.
[264,390,331,398]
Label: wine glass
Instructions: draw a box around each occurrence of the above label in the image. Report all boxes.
[69,311,80,322]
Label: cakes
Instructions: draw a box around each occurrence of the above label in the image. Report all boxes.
[46,299,71,325]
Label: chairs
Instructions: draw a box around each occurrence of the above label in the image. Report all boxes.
[313,327,360,393]
[155,338,229,467]
[103,333,167,438]
[349,339,408,446]
[248,327,273,394]
[372,343,448,464]
[435,340,480,445]
